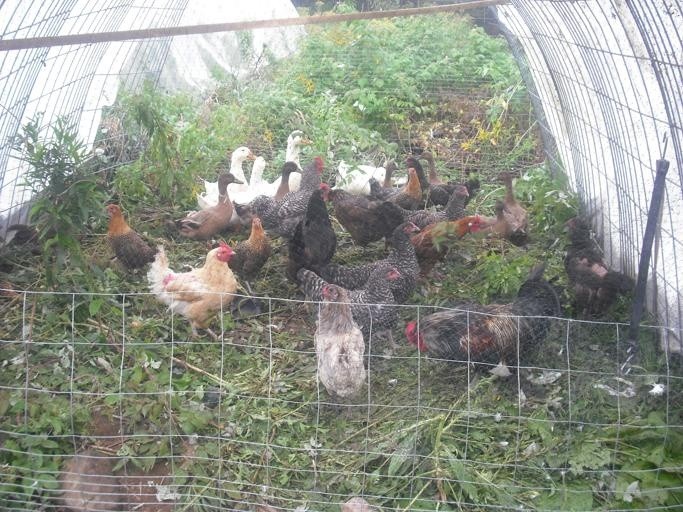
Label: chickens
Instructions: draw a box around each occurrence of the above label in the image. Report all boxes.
[412,212,489,283]
[143,241,242,344]
[105,203,158,282]
[287,181,342,277]
[403,257,565,384]
[358,166,424,215]
[263,155,328,244]
[224,213,277,297]
[562,215,637,333]
[314,219,423,306]
[384,183,473,241]
[294,265,402,357]
[312,283,369,404]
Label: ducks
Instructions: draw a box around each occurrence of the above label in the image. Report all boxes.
[190,145,273,207]
[273,128,313,199]
[483,168,534,245]
[162,171,244,248]
[379,147,480,209]
